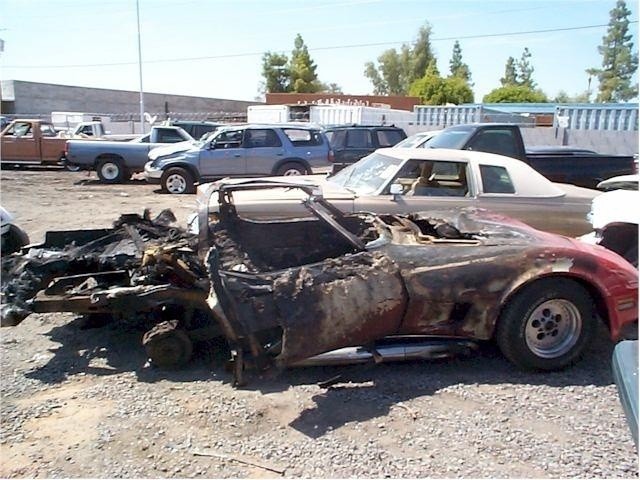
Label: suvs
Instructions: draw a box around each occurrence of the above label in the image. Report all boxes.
[322,124,408,176]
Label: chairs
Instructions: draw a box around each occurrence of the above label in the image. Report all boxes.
[405,177,430,196]
[240,132,252,147]
[456,167,468,196]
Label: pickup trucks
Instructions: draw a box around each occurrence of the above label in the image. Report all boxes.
[391,121,638,189]
[0,117,110,173]
[63,125,197,185]
[73,121,147,143]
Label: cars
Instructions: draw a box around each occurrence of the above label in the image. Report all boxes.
[192,142,602,241]
[25,175,638,381]
[143,122,335,193]
